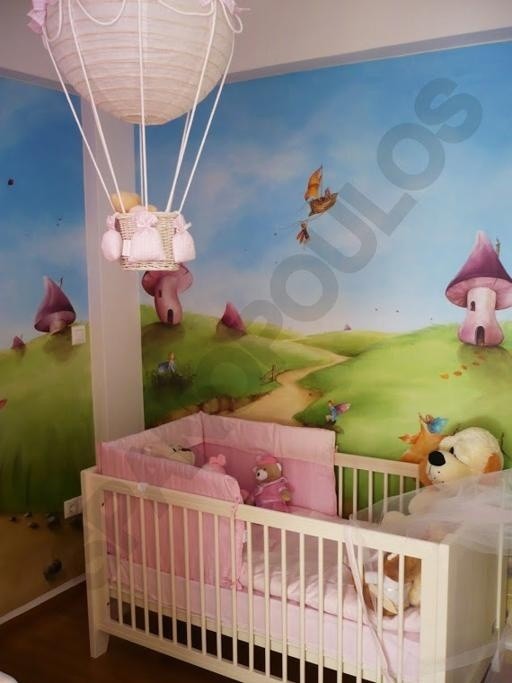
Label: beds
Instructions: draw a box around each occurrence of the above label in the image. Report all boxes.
[80,455,512,683]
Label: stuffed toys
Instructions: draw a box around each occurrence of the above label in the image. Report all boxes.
[245,455,291,514]
[338,425,503,617]
[201,454,227,475]
[143,440,195,466]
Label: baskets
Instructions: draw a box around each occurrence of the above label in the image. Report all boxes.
[115,211,180,271]
